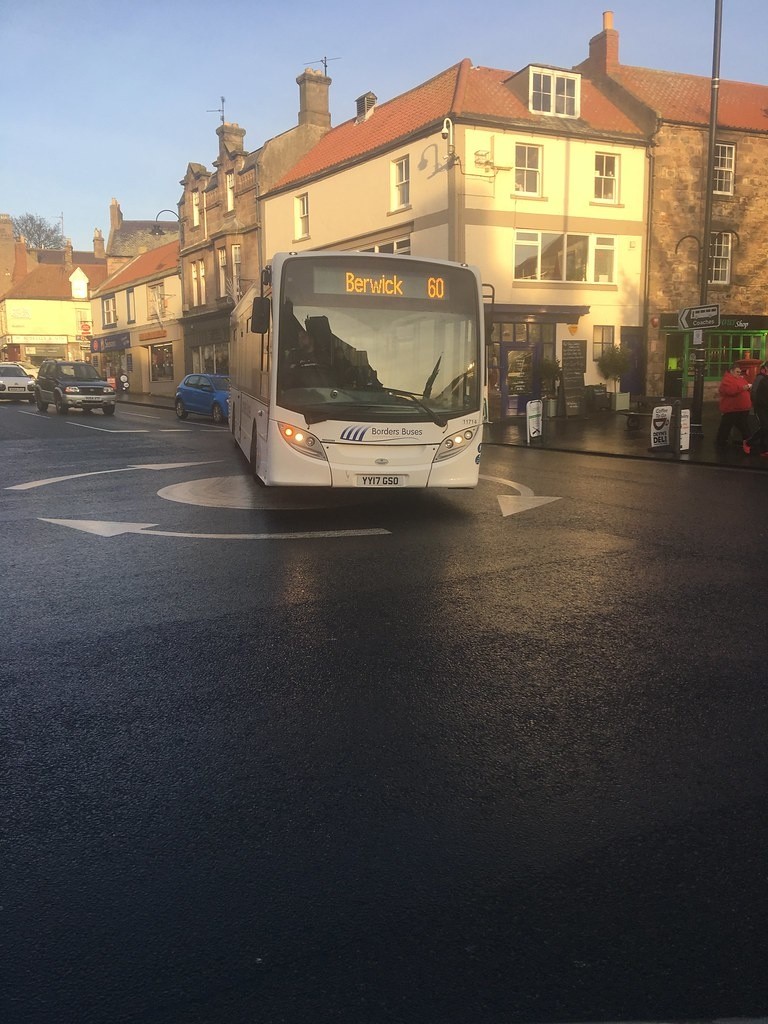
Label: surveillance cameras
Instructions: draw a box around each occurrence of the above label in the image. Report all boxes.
[441,128,449,139]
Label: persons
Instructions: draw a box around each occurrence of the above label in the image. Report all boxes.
[743,361,768,457]
[332,347,357,385]
[716,362,753,450]
[282,325,328,373]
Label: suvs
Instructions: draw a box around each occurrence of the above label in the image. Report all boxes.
[0,362,36,404]
[34,358,117,416]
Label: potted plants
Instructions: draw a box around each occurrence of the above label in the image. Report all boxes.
[597,342,633,411]
[540,355,562,417]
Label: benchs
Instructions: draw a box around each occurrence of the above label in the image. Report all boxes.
[616,394,694,431]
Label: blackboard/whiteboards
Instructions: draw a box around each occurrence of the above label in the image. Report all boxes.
[560,367,586,415]
[562,340,587,373]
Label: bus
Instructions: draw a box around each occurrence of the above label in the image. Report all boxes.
[227,250,495,489]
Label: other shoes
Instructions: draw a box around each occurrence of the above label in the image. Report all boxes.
[743,440,750,453]
[761,452,768,456]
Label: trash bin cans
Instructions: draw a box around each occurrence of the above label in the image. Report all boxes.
[587,383,608,411]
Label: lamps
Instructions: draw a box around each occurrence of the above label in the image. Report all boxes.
[149,209,182,240]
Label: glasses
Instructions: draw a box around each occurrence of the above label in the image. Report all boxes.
[734,369,742,374]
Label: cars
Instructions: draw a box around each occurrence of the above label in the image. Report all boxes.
[174,373,229,425]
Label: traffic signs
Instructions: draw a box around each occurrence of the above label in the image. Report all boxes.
[678,304,721,330]
